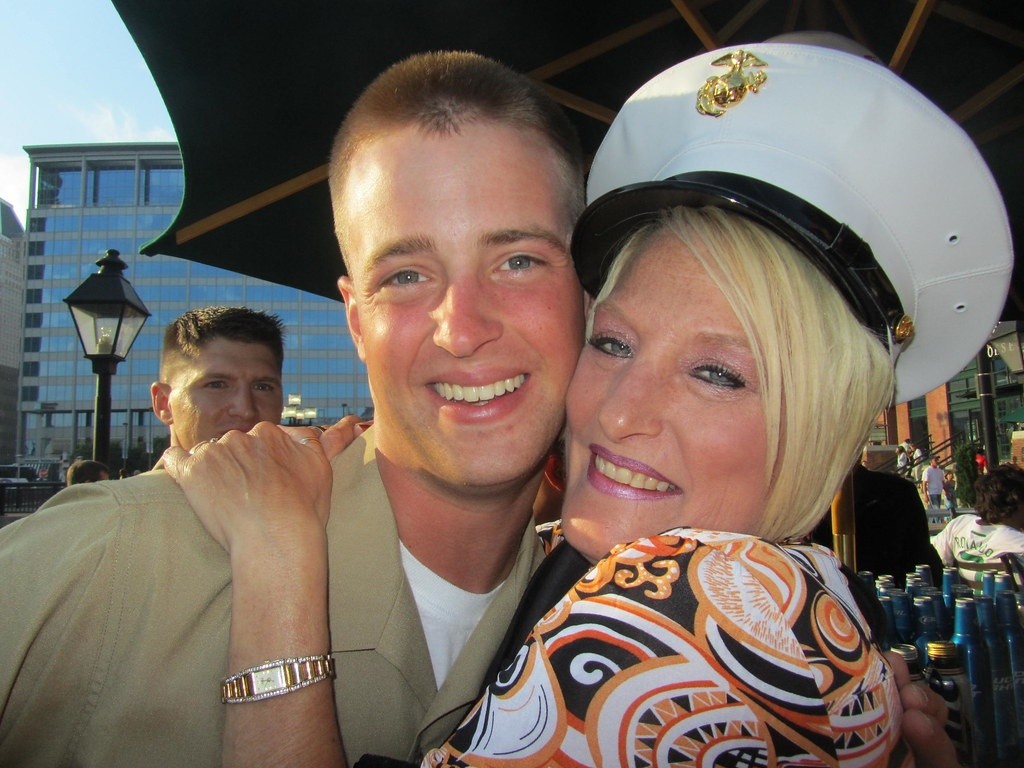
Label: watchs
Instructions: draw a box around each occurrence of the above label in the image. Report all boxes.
[221,656,335,704]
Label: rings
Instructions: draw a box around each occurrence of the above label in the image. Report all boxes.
[193,439,218,454]
[299,437,322,447]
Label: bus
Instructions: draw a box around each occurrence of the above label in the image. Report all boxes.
[923,507,979,536]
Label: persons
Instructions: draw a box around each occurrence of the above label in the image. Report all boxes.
[922,453,945,536]
[975,448,990,475]
[67,460,140,490]
[896,446,907,479]
[163,39,1014,768]
[0,53,956,768]
[812,464,936,593]
[150,306,284,453]
[942,471,958,524]
[900,439,914,477]
[910,442,923,482]
[932,461,1024,606]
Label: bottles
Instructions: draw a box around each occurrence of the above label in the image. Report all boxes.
[857,565,1024,768]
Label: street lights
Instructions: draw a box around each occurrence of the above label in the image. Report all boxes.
[341,402,348,417]
[60,248,154,463]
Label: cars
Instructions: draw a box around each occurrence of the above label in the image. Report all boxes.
[0,464,37,483]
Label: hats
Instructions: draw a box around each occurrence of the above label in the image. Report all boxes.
[564,40,1014,406]
[929,455,942,461]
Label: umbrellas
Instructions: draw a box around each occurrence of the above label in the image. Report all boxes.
[112,0,1024,576]
